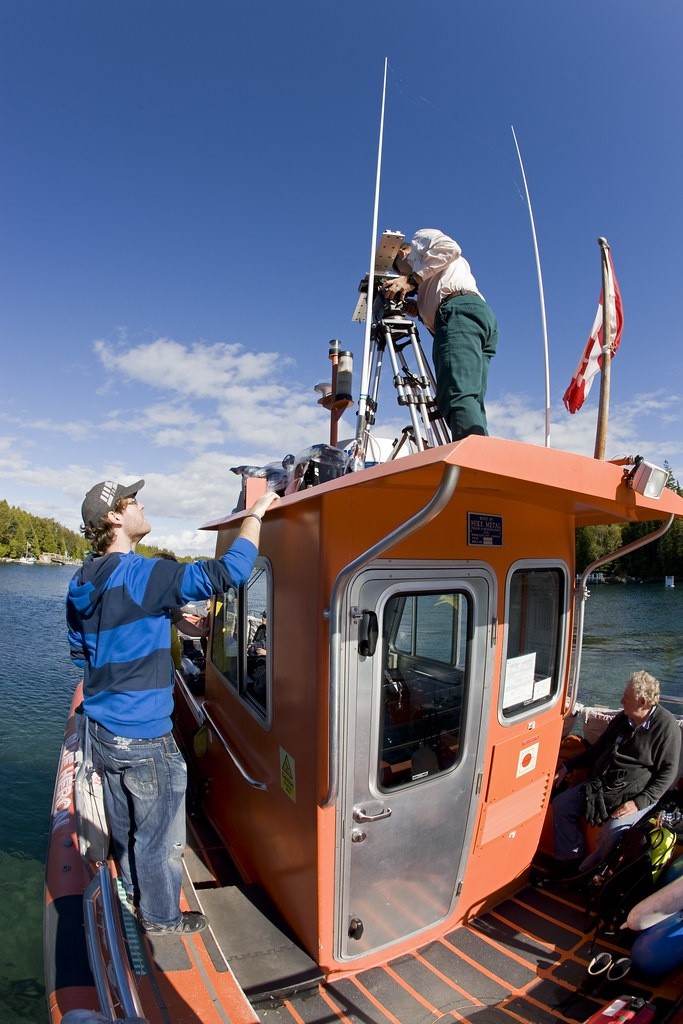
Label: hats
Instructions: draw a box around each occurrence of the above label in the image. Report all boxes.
[81,480,146,532]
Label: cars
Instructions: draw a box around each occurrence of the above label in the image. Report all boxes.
[674,575,683,581]
[631,575,641,581]
[647,575,664,582]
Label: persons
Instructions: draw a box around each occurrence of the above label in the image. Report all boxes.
[381,229,497,440]
[212,601,231,671]
[554,671,681,895]
[201,599,211,656]
[253,609,267,656]
[394,746,441,785]
[66,478,280,935]
[150,553,209,667]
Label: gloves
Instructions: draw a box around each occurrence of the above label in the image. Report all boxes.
[596,768,629,793]
[583,781,609,827]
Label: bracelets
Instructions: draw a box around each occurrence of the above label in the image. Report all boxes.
[245,513,262,525]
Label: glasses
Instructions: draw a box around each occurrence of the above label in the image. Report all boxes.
[392,248,407,273]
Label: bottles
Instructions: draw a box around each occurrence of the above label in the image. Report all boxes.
[342,450,364,475]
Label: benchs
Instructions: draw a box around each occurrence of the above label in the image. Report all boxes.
[538,798,683,873]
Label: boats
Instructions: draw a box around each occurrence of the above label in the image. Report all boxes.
[21,553,37,562]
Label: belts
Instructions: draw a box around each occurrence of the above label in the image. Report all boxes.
[443,289,477,302]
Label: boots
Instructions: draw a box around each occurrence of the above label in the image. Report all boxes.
[578,952,632,1002]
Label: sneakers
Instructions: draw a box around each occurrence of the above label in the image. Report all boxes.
[140,911,209,936]
[126,891,137,905]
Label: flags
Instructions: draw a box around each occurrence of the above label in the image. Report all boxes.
[563,249,624,413]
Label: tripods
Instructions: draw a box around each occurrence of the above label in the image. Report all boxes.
[361,315,452,468]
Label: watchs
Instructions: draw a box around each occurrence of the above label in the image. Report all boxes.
[408,274,419,287]
